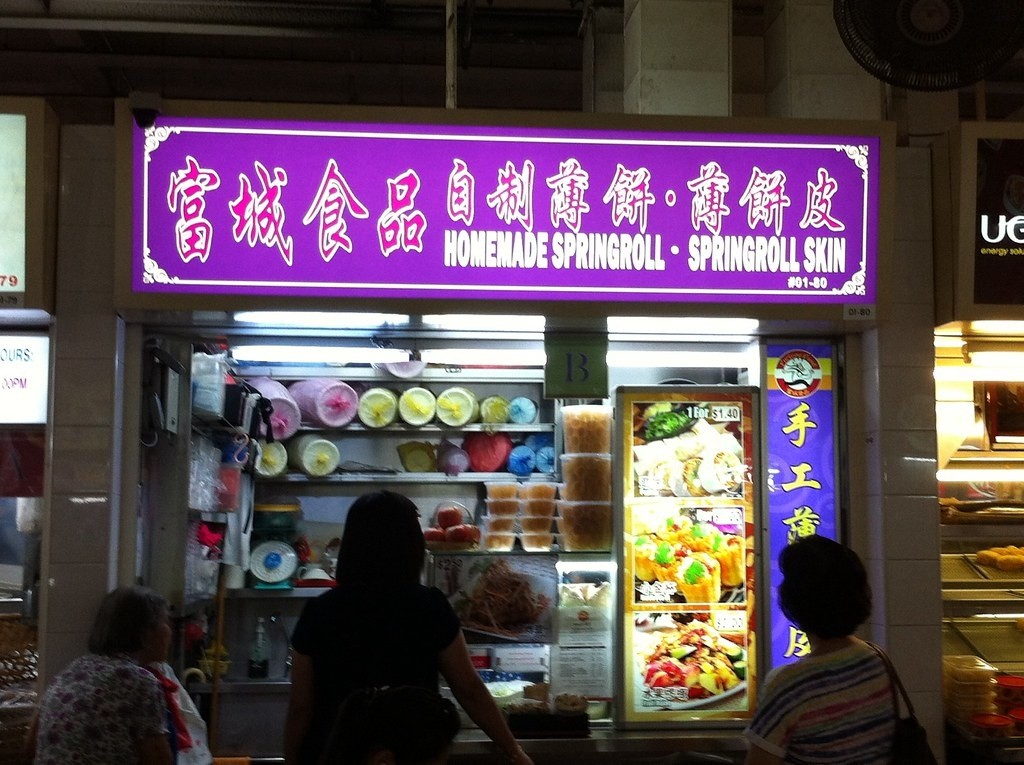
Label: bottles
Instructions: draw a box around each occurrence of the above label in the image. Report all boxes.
[177,664,205,688]
[247,617,270,680]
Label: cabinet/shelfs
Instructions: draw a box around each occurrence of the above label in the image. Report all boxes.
[190,366,555,695]
[936,434,1024,765]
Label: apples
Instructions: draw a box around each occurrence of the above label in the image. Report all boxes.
[422,506,480,543]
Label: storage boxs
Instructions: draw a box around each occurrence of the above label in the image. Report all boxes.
[946,689,997,708]
[944,672,998,695]
[947,699,998,720]
[943,654,999,682]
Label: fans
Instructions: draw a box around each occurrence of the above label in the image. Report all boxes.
[833,0,1024,93]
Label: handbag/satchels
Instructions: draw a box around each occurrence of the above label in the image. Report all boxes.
[864,640,937,765]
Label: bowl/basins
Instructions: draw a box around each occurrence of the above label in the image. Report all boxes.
[554,404,615,551]
[517,481,557,552]
[584,700,608,721]
[481,480,518,552]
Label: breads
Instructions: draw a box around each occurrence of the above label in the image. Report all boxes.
[976,545,1024,572]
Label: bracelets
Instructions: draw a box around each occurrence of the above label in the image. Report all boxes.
[508,744,522,763]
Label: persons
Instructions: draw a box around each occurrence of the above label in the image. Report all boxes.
[20,584,176,765]
[740,532,901,765]
[280,490,538,764]
